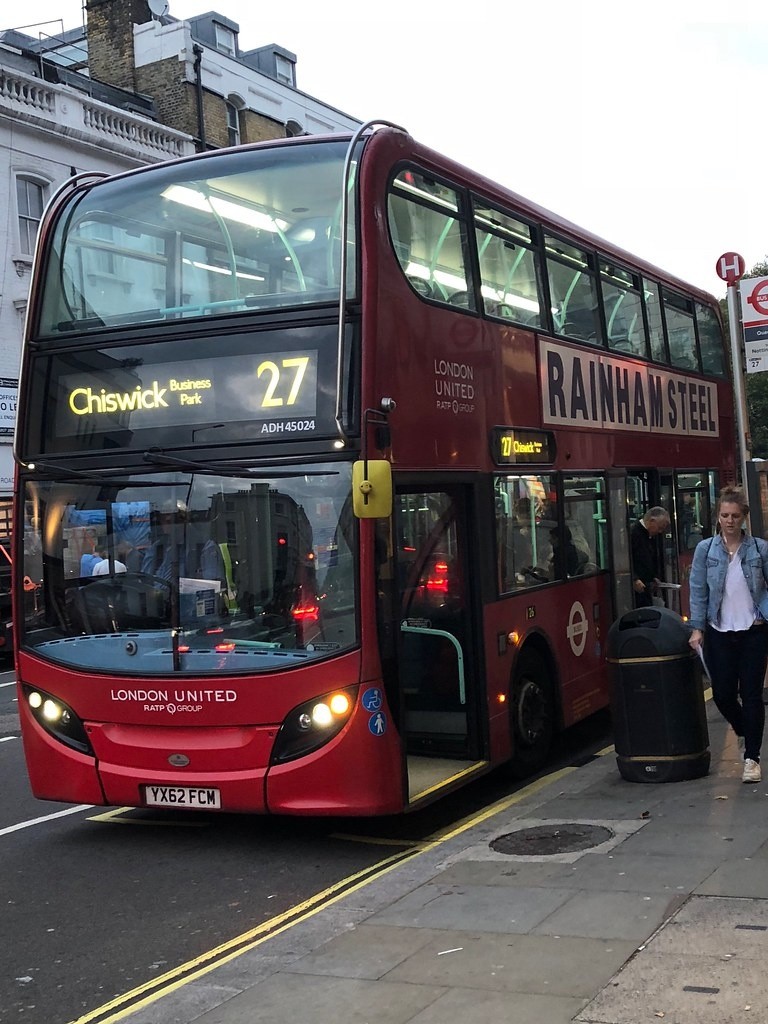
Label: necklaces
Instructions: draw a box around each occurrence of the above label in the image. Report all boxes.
[729,551,734,556]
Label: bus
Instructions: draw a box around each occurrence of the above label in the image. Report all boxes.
[11,118,754,819]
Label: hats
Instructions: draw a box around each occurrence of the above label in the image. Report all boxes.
[513,497,531,513]
[550,523,571,540]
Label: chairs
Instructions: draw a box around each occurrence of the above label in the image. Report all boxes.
[408,274,695,371]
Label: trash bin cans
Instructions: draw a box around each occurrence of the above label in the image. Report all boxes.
[598,604,710,785]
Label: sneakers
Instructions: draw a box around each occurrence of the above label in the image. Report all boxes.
[737,736,746,753]
[741,758,762,782]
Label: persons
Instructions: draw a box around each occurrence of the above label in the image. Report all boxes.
[688,486,768,782]
[514,482,705,587]
[24,497,353,627]
[630,507,670,609]
